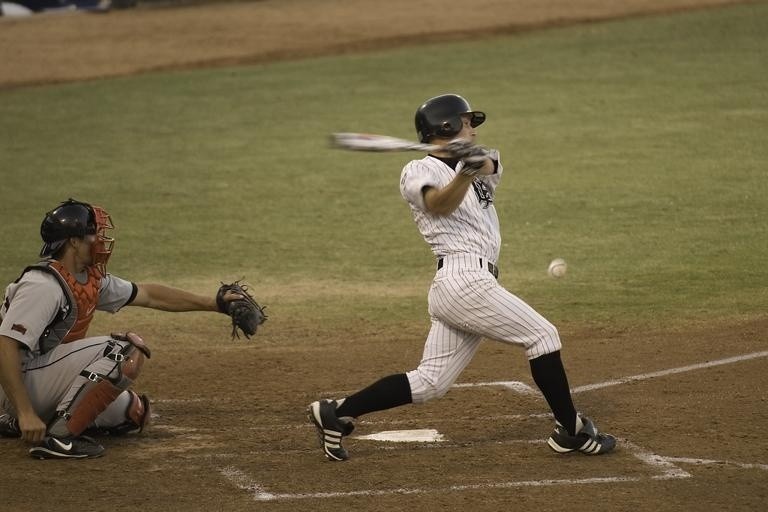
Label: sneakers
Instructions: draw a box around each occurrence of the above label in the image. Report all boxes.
[548,417,616,456]
[309,399,355,461]
[30,432,103,461]
[0,413,22,438]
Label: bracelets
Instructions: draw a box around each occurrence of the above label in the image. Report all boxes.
[460,165,477,179]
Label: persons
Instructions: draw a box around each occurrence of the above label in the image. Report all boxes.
[0,196,268,460]
[306,93,618,464]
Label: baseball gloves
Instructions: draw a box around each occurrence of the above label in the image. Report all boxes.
[216,283,267,335]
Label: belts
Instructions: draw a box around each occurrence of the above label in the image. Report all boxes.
[438,254,499,278]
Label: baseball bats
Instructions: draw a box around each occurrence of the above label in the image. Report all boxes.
[328,130,485,161]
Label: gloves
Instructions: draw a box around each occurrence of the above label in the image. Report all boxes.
[445,139,489,176]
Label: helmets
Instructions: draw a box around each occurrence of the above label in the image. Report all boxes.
[40,198,115,278]
[413,93,487,142]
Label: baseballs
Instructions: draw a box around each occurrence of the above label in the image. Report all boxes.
[547,258,569,278]
